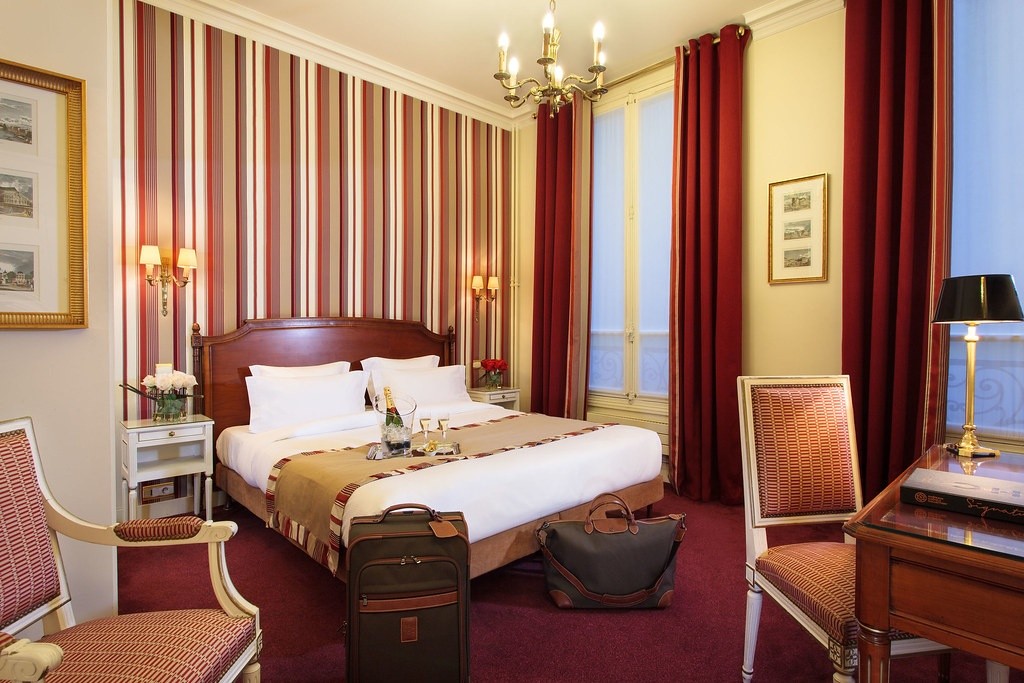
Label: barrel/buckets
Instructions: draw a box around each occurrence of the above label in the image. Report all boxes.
[373,392,417,452]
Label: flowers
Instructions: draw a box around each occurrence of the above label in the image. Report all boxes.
[140,370,198,422]
[481,359,509,388]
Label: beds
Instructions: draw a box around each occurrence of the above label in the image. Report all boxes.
[192,317,664,639]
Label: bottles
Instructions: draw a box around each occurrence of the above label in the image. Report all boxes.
[383,387,410,454]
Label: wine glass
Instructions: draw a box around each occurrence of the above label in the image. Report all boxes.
[437,413,449,441]
[419,410,431,444]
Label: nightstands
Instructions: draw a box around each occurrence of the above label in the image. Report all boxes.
[120,413,215,523]
[465,387,520,412]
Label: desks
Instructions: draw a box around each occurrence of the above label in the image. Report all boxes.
[842,444,1024,683]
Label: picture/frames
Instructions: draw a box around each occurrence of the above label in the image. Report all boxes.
[0,57,88,330]
[768,173,828,284]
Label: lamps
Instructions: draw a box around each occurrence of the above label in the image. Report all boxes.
[493,0,608,119]
[472,276,499,323]
[931,274,1024,458]
[139,245,197,317]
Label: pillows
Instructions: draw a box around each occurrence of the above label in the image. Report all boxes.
[372,365,473,419]
[360,355,440,403]
[249,361,351,376]
[245,370,370,433]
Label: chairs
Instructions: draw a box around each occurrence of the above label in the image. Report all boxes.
[0,417,262,683]
[742,375,1010,683]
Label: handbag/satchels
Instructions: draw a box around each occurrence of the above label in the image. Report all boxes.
[535,492,686,610]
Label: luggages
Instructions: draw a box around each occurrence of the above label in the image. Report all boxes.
[344,503,470,683]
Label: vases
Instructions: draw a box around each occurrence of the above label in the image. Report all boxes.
[153,396,187,422]
[484,375,502,390]
